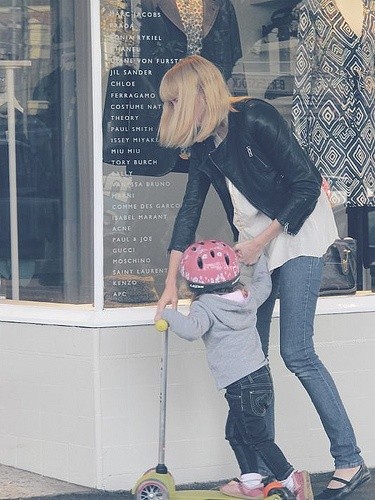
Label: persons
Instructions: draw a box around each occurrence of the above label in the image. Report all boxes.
[125,0,248,177]
[157,55,371,500]
[154,240,314,500]
[291,0,375,209]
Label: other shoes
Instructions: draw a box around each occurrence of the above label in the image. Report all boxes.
[292,471,314,500]
[220,478,265,499]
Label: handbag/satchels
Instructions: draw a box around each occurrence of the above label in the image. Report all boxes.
[262,7,299,73]
[277,236,357,298]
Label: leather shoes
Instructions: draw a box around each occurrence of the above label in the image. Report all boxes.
[314,464,371,499]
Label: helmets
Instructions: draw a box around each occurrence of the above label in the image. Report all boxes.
[178,240,241,293]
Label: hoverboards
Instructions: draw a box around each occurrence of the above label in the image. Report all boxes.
[130,319,295,500]
